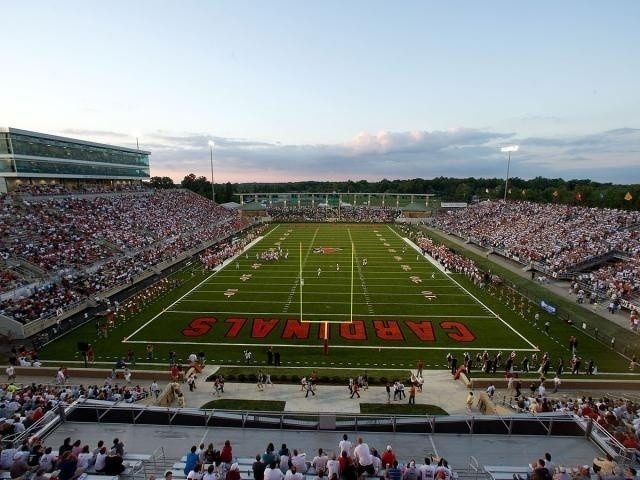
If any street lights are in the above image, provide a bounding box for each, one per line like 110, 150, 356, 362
500, 145, 519, 203
206, 139, 216, 202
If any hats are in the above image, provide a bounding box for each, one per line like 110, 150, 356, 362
386, 445, 393, 452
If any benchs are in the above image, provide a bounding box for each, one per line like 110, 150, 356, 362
155, 455, 329, 480
361, 462, 454, 480
484, 464, 601, 480
2, 449, 165, 480
